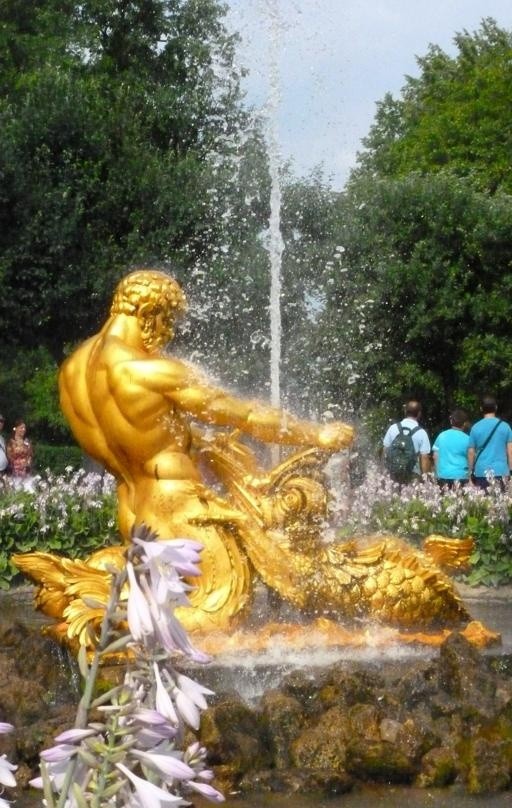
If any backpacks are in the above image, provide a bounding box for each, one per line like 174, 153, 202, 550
385, 422, 423, 483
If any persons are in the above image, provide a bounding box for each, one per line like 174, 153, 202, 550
17, 265, 352, 639
467, 394, 512, 493
0, 414, 10, 474
380, 398, 430, 486
430, 410, 472, 490
7, 420, 36, 476
